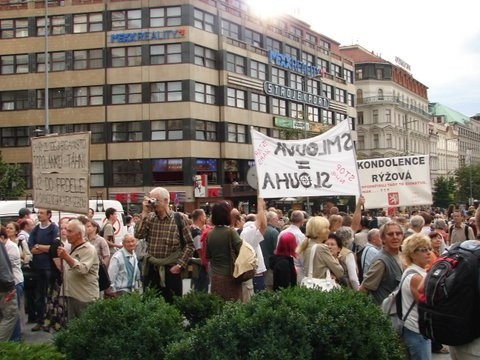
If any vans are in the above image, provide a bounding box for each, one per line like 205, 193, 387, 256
0, 198, 127, 291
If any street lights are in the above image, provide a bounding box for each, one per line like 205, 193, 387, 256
405, 114, 418, 155
302, 73, 321, 216
445, 137, 458, 190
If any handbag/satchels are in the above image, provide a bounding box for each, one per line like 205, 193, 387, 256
180, 257, 199, 279
300, 243, 336, 293
98, 264, 110, 291
229, 229, 254, 282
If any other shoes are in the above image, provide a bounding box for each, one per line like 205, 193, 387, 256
28, 314, 38, 323
31, 325, 41, 331
438, 347, 449, 354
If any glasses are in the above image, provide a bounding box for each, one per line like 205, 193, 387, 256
385, 231, 403, 236
416, 248, 432, 252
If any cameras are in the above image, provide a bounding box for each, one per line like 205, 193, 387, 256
147, 198, 156, 205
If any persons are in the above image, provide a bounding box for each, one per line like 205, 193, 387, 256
230, 198, 310, 291
205, 200, 258, 302
105, 234, 142, 298
301, 193, 406, 305
403, 202, 480, 272
134, 187, 196, 304
381, 233, 432, 360
99, 207, 123, 257
418, 203, 480, 360
88, 208, 94, 218
0, 207, 61, 345
57, 215, 110, 320
121, 212, 146, 236
181, 209, 215, 293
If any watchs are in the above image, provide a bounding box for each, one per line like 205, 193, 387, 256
35, 244, 39, 249
177, 264, 182, 268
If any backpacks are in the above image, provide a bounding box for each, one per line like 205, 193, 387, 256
382, 270, 422, 336
424, 240, 480, 346
